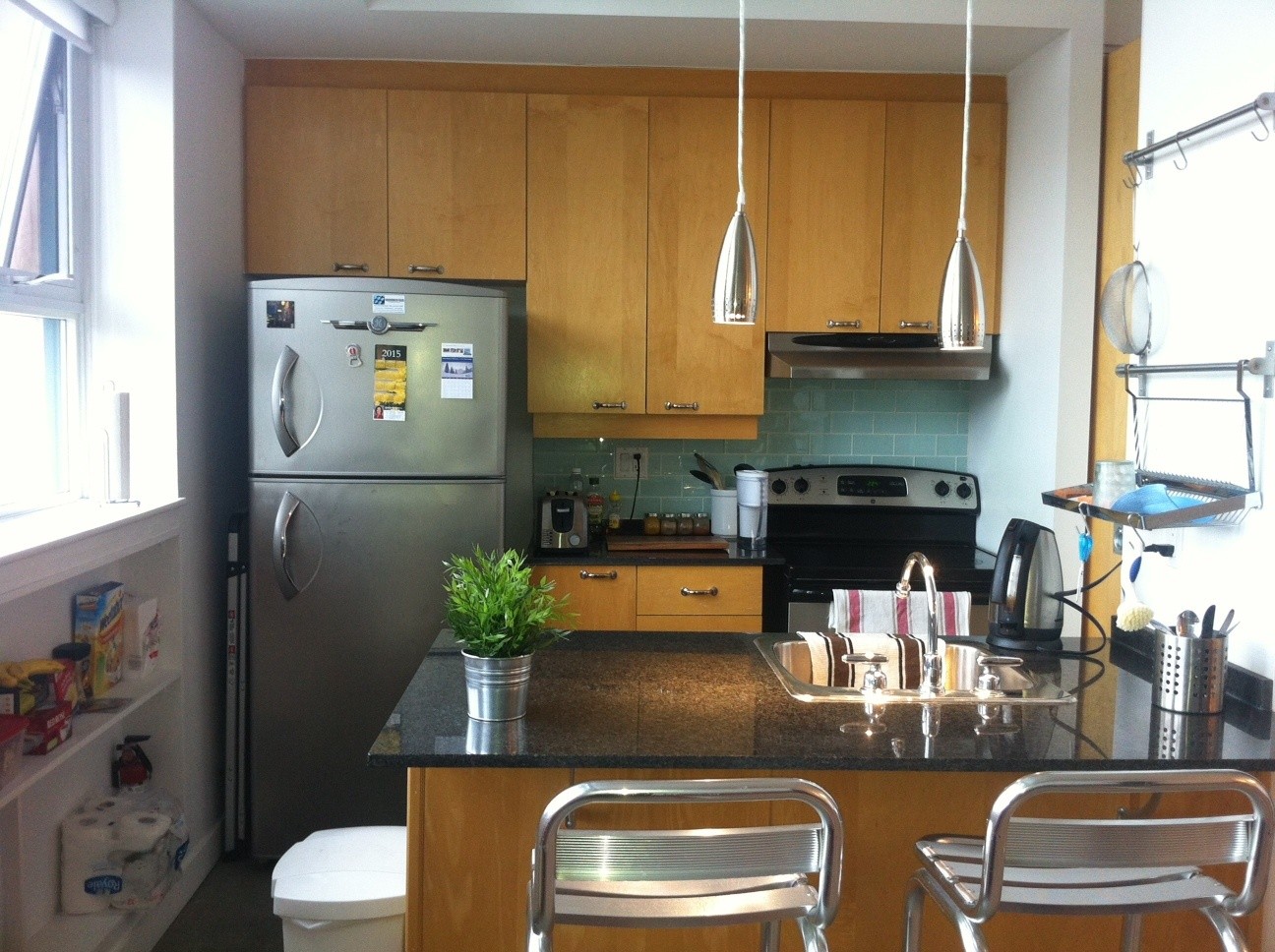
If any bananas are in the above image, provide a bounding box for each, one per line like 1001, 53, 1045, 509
0, 660, 66, 690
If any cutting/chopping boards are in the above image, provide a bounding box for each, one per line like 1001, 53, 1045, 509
607, 536, 729, 551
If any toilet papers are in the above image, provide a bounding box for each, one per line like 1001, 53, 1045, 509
58, 794, 171, 915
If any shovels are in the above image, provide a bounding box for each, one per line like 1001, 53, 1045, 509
695, 453, 723, 490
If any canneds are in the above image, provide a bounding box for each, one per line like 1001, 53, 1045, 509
645, 512, 709, 536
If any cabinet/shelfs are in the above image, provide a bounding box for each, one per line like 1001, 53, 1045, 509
0, 668, 183, 952
635, 566, 764, 633
764, 71, 1006, 334
243, 58, 527, 284
525, 65, 769, 440
521, 565, 636, 632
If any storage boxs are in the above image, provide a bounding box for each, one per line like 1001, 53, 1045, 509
123, 592, 160, 682
24, 700, 73, 756
0, 714, 30, 789
75, 581, 124, 699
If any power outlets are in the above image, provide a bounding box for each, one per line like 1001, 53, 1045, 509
1164, 527, 1183, 562
613, 447, 648, 479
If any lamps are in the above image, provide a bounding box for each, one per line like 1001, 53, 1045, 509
937, 1, 985, 351
711, 0, 759, 325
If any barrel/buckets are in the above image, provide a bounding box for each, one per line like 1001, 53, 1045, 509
460, 648, 535, 721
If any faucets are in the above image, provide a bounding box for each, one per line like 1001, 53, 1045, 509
896, 552, 945, 698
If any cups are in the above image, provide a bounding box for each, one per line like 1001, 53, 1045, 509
65, 715, 73, 728
710, 487, 737, 538
1091, 461, 1136, 509
59, 724, 70, 742
11, 739, 31, 754
25, 732, 45, 748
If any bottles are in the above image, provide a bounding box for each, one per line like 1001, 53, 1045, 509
660, 513, 676, 535
606, 489, 622, 536
643, 513, 660, 535
569, 468, 583, 495
52, 643, 95, 712
676, 513, 694, 535
586, 478, 604, 536
693, 513, 710, 535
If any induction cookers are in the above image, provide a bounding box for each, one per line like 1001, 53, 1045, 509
763, 463, 998, 581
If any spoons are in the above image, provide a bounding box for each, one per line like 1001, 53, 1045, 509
1149, 610, 1200, 638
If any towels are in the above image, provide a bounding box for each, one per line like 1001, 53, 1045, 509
828, 588, 973, 636
796, 631, 946, 688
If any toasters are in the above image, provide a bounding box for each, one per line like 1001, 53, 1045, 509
539, 490, 589, 555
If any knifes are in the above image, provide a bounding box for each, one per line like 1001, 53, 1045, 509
1202, 605, 1234, 638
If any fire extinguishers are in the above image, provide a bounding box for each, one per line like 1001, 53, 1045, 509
111, 733, 154, 791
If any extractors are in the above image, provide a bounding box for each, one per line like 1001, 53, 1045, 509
766, 332, 992, 381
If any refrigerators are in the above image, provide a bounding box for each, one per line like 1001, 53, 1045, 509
248, 277, 506, 868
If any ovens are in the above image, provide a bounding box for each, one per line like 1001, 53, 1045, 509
762, 582, 990, 637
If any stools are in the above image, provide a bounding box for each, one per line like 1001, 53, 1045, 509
902, 767, 1274, 952
526, 778, 845, 952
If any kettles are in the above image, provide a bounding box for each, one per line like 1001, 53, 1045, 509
986, 518, 1064, 651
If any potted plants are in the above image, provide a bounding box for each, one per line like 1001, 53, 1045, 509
440, 541, 581, 721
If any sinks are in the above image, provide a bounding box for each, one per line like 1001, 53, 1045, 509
753, 633, 1078, 704
